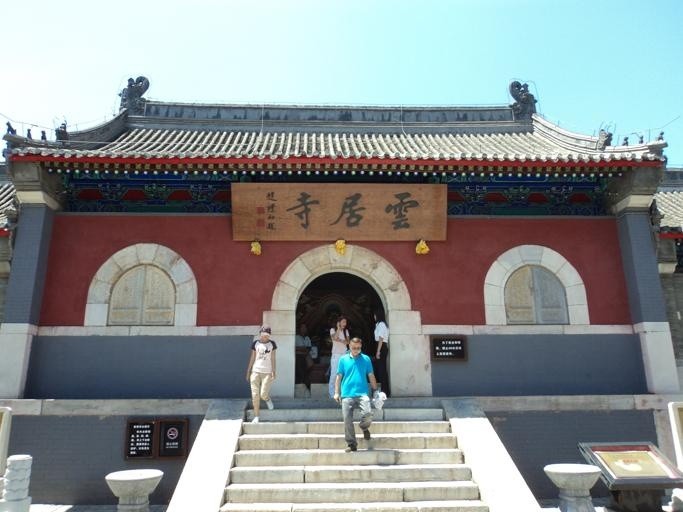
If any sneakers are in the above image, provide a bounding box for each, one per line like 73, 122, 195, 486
363, 430, 370, 440
266, 399, 273, 409
345, 443, 357, 451
251, 416, 259, 423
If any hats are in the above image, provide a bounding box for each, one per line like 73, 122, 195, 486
260, 326, 270, 336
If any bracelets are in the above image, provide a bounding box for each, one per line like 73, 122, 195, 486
374, 389, 378, 391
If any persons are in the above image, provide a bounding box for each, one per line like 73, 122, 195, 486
328, 315, 350, 400
373, 308, 389, 397
246, 327, 277, 423
334, 337, 379, 453
296, 323, 312, 390
